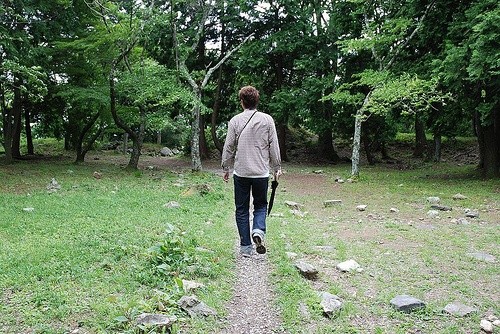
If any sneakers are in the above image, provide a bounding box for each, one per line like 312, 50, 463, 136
252, 232, 267, 254
240, 244, 252, 258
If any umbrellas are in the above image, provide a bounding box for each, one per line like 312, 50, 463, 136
268, 170, 279, 216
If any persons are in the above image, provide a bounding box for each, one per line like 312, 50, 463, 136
221, 85, 281, 256
